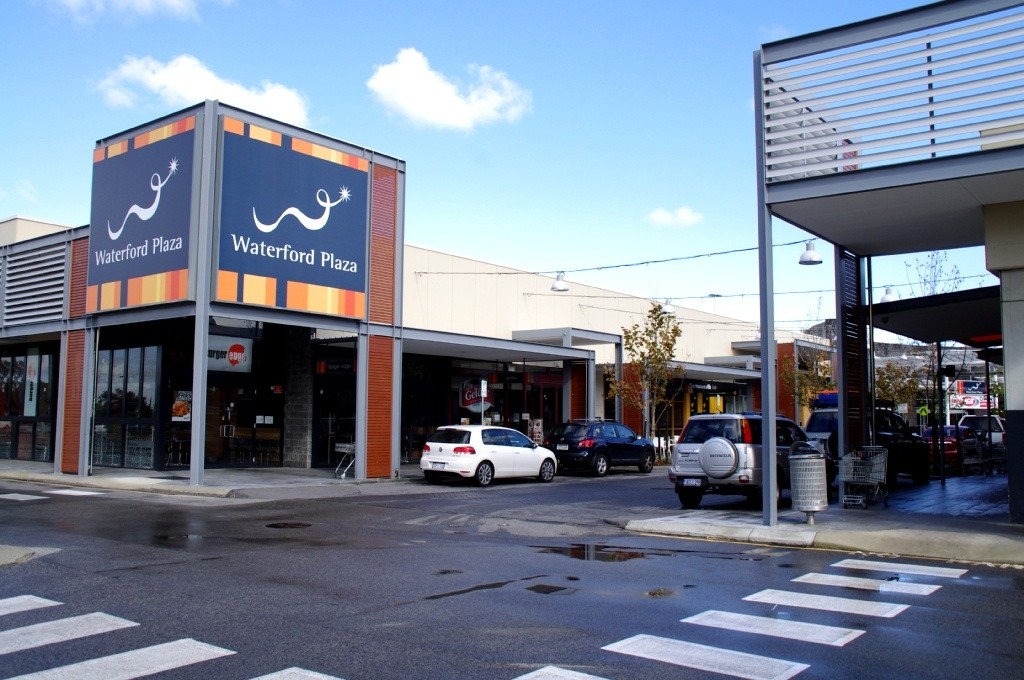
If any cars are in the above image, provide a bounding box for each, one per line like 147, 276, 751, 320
419, 424, 557, 486
922, 425, 986, 465
958, 414, 1005, 452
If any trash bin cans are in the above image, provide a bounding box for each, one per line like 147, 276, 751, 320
789, 440, 828, 512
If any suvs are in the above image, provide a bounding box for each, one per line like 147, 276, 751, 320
802, 396, 931, 487
669, 411, 814, 506
542, 418, 657, 478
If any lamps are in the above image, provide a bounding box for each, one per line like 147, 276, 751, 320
899, 352, 908, 359
755, 331, 762, 340
660, 299, 675, 314
798, 240, 822, 265
881, 287, 899, 302
551, 273, 569, 291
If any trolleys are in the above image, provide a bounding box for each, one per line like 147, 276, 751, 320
333, 444, 355, 479
